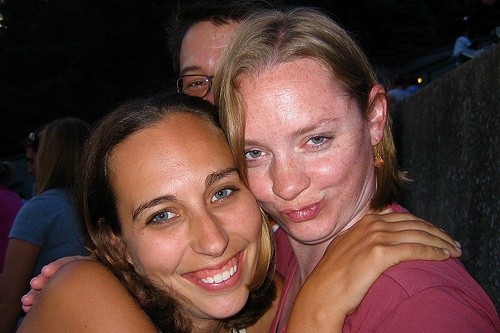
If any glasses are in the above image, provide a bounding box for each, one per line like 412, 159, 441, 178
177, 74, 214, 98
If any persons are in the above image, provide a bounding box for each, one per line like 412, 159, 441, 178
20, 6, 500, 333
174, 5, 253, 107
0, 118, 98, 333
17, 91, 464, 333
